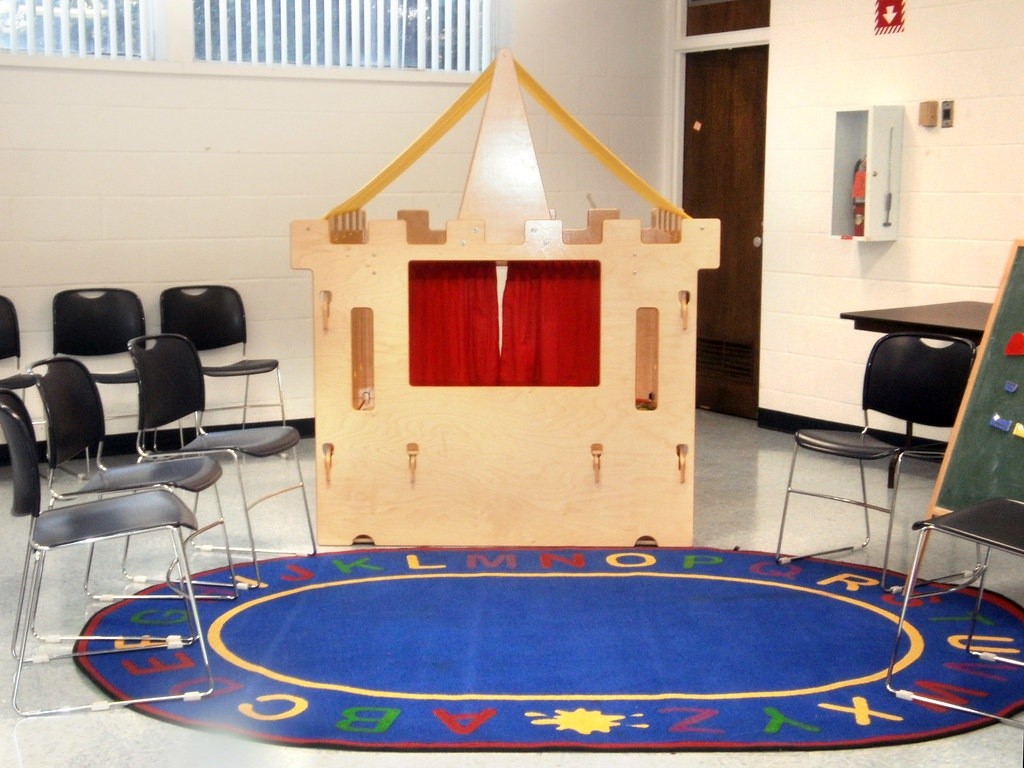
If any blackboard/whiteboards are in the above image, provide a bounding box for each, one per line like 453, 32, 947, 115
929, 236, 1024, 518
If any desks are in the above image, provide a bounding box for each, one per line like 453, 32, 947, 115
839, 300, 993, 508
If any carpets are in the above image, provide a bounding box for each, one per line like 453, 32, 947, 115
72, 546, 1024, 755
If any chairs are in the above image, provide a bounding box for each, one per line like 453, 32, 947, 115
884, 496, 1024, 729
159, 284, 296, 462
52, 288, 157, 482
774, 330, 983, 593
0, 389, 213, 718
0, 296, 57, 485
24, 357, 238, 642
120, 333, 317, 592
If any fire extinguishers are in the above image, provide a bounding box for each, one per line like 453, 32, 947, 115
853, 205, 865, 236
